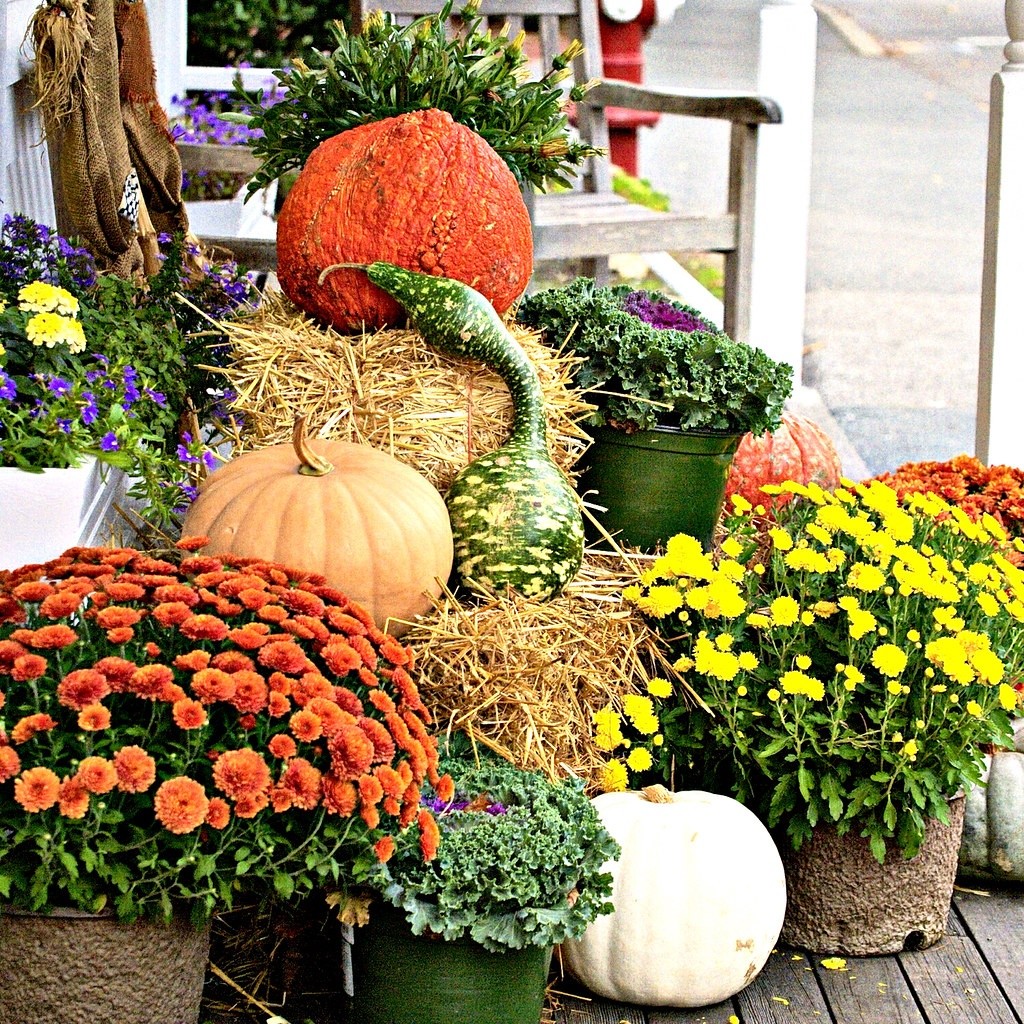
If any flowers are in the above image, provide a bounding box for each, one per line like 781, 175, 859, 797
0, 0, 1024, 932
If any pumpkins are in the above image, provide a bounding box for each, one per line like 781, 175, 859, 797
952, 716, 1024, 881
278, 106, 535, 335
552, 783, 786, 1007
717, 411, 841, 523
178, 410, 452, 636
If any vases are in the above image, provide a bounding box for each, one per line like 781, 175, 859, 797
774, 775, 967, 959
0, 894, 213, 1024
341, 878, 553, 1024
577, 411, 747, 552
177, 140, 268, 175
0, 437, 149, 586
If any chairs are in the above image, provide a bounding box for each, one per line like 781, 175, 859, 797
356, 0, 786, 348
